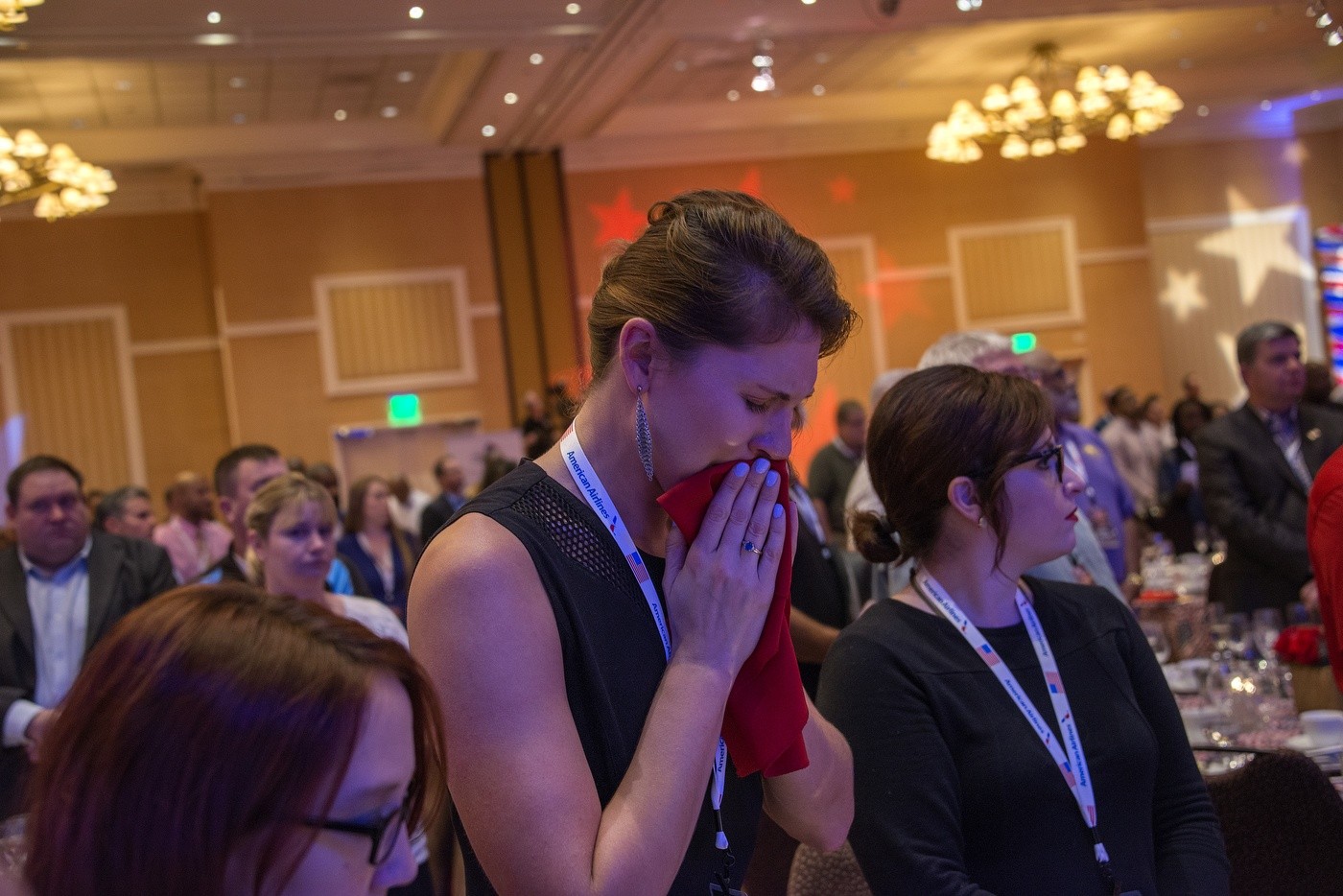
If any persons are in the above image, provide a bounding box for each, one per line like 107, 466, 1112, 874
13, 579, 432, 894
847, 328, 1136, 620
806, 397, 870, 539
0, 372, 585, 758
1016, 316, 1341, 613
398, 183, 867, 895
812, 363, 1222, 896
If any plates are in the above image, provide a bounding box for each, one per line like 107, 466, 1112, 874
1288, 735, 1342, 756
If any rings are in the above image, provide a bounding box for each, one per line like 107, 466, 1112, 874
741, 540, 762, 556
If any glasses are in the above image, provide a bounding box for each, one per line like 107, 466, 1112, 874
254, 780, 421, 866
930, 444, 1065, 512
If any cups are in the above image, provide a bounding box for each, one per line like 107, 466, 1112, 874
1136, 543, 1302, 777
1301, 708, 1341, 747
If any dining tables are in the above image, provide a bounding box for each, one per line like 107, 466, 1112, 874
1139, 643, 1343, 796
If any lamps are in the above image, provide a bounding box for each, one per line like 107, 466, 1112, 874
0, 125, 122, 225
920, 45, 1185, 167
